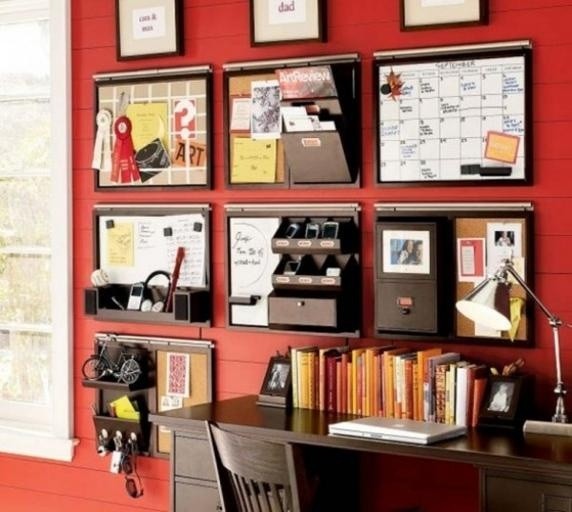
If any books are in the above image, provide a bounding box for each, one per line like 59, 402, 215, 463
291, 345, 492, 430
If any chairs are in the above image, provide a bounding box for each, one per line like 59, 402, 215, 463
204, 417, 305, 512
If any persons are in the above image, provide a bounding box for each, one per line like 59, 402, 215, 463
271, 363, 283, 391
397, 241, 414, 264
487, 383, 508, 412
497, 231, 510, 246
408, 241, 422, 264
253, 88, 276, 118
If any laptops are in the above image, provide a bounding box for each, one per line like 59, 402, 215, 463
328, 416, 466, 446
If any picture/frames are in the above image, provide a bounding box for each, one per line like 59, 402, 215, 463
255, 355, 291, 397
479, 370, 520, 420
246, 0, 330, 46
369, 198, 539, 348
150, 342, 213, 461
371, 38, 536, 188
398, 0, 490, 33
220, 200, 367, 341
90, 200, 215, 330
220, 51, 366, 192
88, 62, 218, 194
112, 0, 185, 60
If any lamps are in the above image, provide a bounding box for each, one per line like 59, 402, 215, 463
451, 256, 572, 437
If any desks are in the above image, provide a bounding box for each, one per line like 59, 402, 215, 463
148, 396, 572, 512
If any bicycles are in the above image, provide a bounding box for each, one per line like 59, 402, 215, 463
81, 335, 142, 388
